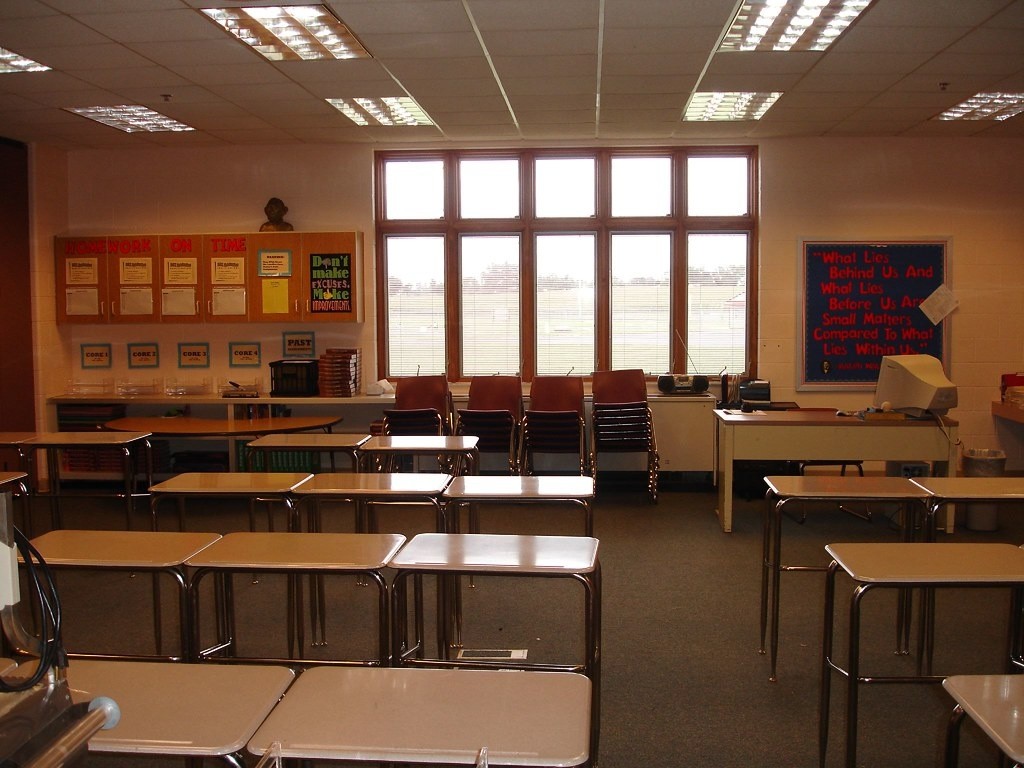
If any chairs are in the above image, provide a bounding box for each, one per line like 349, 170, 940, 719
775, 408, 871, 524
370, 368, 659, 504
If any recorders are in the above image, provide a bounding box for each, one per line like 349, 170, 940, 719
657, 329, 710, 394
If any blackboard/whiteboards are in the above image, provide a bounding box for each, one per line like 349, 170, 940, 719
798, 238, 951, 388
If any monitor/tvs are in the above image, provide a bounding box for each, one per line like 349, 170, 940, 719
873, 354, 958, 419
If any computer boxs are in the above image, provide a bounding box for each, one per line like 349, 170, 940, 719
882, 460, 931, 527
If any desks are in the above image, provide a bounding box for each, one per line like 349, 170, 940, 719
759, 476, 1024, 768
713, 408, 959, 534
0, 431, 602, 768
52, 393, 718, 495
106, 416, 342, 508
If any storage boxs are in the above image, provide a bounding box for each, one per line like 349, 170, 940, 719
63, 376, 264, 395
269, 348, 362, 398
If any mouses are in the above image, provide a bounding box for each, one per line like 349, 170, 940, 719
836, 411, 852, 416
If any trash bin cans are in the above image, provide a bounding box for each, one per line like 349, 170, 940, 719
961, 447, 1008, 532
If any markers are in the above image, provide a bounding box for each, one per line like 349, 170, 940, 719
229, 381, 246, 391
